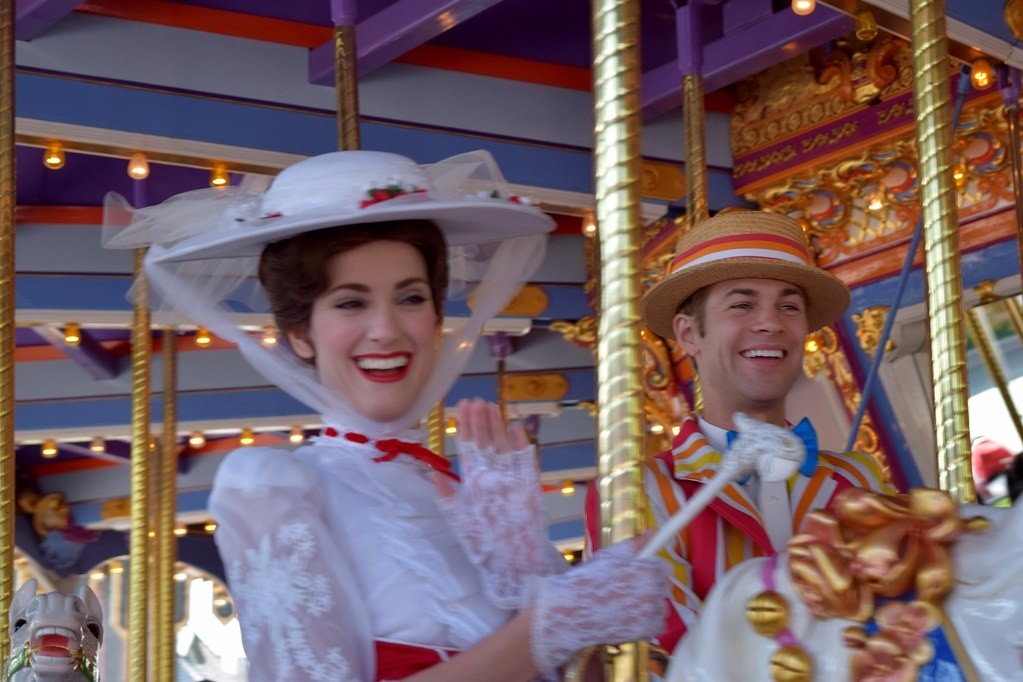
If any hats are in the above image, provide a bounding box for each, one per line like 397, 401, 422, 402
149, 150, 556, 267
641, 212, 851, 343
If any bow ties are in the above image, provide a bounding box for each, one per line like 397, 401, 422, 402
725, 417, 818, 484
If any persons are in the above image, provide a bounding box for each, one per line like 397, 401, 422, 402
207, 151, 674, 682
584, 210, 982, 682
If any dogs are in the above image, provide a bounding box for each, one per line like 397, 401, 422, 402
6, 579, 105, 681
